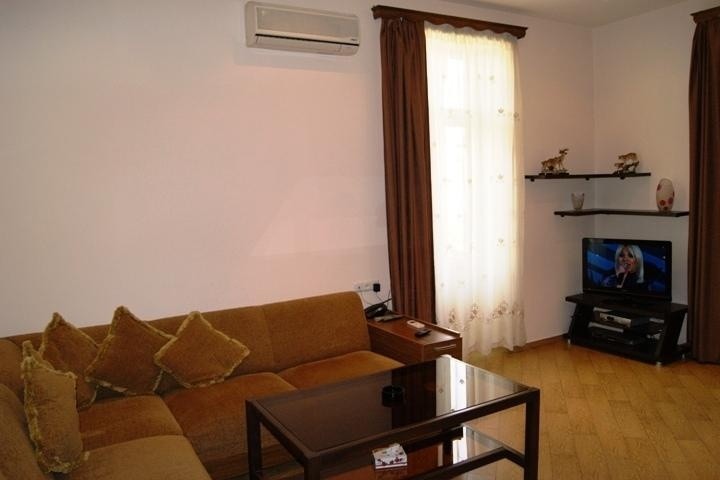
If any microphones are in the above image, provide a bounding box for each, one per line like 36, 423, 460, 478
617, 264, 628, 288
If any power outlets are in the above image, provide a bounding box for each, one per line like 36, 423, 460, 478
354, 280, 379, 292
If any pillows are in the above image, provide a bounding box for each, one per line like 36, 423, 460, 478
20, 306, 250, 472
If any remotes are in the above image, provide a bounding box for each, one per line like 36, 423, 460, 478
415, 329, 431, 337
408, 320, 425, 328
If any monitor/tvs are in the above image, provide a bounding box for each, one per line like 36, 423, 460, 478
582, 237, 672, 304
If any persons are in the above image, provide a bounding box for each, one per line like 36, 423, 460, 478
600, 243, 656, 291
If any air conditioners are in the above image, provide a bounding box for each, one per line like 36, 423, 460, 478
246, 0, 360, 57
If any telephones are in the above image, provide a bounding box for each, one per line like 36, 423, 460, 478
364, 297, 393, 319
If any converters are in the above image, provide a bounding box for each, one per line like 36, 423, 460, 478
373, 283, 380, 292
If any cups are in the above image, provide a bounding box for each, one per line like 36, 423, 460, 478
571, 192, 585, 209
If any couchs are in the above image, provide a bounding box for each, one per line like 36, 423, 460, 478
0, 290, 403, 480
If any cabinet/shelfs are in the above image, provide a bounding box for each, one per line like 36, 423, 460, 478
367, 312, 462, 367
563, 297, 688, 365
525, 172, 690, 217
